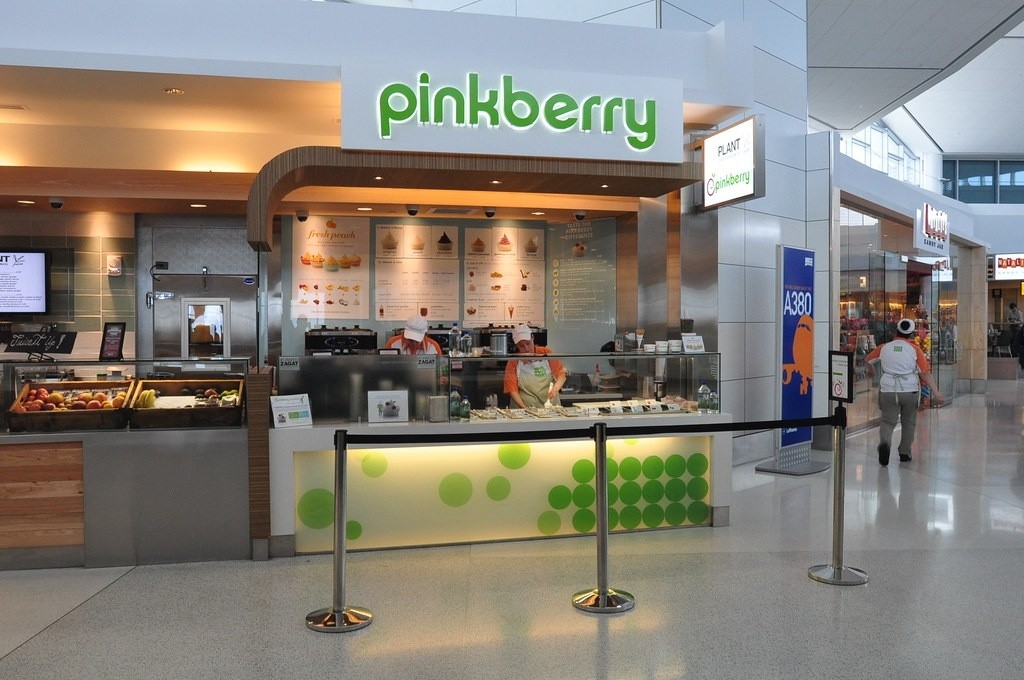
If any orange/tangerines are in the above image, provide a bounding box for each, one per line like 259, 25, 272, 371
102, 391, 126, 408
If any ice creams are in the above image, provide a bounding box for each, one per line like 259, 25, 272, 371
508, 304, 514, 320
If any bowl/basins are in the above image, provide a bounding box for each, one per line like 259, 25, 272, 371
643, 344, 656, 355
668, 339, 682, 353
655, 341, 668, 354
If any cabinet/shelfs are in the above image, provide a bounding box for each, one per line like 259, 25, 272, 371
841, 329, 872, 394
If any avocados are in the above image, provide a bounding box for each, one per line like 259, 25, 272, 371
154, 387, 204, 408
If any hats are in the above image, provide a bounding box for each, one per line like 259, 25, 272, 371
512, 325, 532, 343
404, 315, 428, 343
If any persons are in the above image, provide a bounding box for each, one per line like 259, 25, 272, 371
1007, 303, 1024, 357
503, 325, 567, 409
385, 314, 450, 389
864, 319, 944, 466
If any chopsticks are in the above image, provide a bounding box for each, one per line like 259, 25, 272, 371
681, 319, 694, 333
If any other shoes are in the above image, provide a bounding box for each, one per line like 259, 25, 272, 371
898, 454, 912, 462
879, 442, 890, 465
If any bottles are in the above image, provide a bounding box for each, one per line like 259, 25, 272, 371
451, 387, 462, 422
459, 395, 470, 421
697, 382, 711, 413
460, 330, 472, 357
449, 323, 461, 356
708, 389, 718, 414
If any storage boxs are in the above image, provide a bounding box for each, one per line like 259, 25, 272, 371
7, 380, 135, 431
127, 380, 244, 431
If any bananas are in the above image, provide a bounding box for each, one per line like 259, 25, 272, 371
138, 388, 155, 408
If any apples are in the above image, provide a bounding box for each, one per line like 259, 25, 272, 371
22, 388, 105, 411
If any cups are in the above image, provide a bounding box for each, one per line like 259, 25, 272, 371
654, 381, 664, 399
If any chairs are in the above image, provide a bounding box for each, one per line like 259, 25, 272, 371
992, 330, 1012, 357
